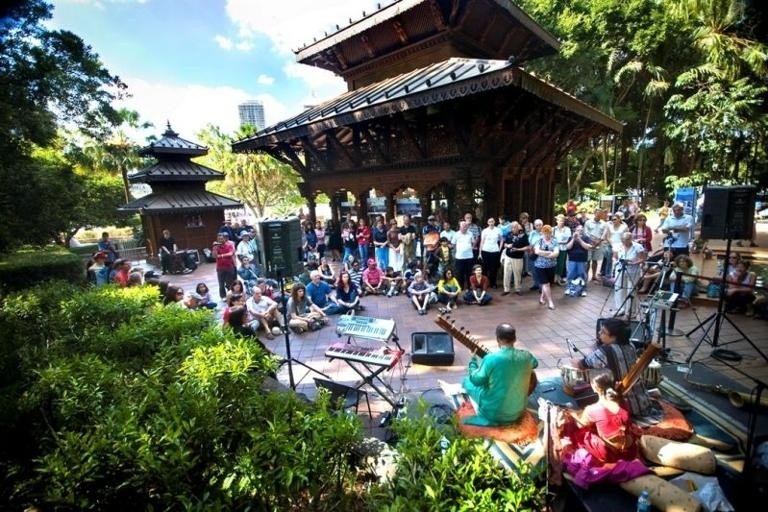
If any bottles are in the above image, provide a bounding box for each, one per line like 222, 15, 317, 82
728, 265, 738, 280
636, 491, 652, 511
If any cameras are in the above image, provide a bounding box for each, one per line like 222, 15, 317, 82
618, 259, 625, 263
215, 243, 223, 246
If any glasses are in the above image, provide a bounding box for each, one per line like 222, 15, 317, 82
175, 291, 184, 297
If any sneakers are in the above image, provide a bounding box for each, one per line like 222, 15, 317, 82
678, 301, 691, 309
498, 273, 602, 297
222, 289, 429, 340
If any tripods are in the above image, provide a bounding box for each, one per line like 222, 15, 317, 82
599, 264, 643, 319
631, 262, 712, 358
686, 240, 768, 363
272, 278, 331, 391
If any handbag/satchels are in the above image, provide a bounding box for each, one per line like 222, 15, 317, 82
527, 244, 539, 262
706, 280, 729, 298
500, 249, 509, 265
569, 277, 586, 298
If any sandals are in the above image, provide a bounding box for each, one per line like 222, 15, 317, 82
438, 306, 448, 315
538, 296, 556, 311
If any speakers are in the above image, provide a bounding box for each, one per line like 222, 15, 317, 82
596, 318, 653, 348
700, 184, 756, 240
258, 216, 305, 279
409, 332, 454, 364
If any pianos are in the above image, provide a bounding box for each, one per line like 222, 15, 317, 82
322, 313, 406, 368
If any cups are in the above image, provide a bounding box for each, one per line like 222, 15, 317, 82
715, 259, 722, 269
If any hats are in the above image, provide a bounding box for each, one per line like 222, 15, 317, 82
240, 230, 250, 238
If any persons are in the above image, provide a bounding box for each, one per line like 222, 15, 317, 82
229, 305, 277, 381
92, 229, 218, 309
569, 315, 649, 413
468, 322, 538, 422
639, 200, 699, 308
212, 200, 652, 332
712, 249, 756, 313
566, 367, 636, 463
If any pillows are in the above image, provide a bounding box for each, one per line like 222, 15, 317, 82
621, 397, 692, 441
641, 435, 716, 475
648, 464, 684, 476
456, 402, 536, 445
685, 433, 732, 450
712, 451, 745, 474
621, 470, 702, 511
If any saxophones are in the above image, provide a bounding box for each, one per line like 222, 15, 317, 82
684, 359, 768, 408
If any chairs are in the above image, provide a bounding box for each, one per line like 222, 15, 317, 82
314, 377, 373, 420
